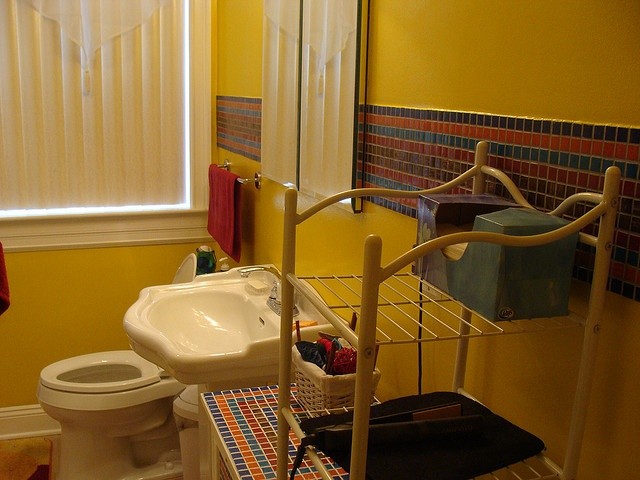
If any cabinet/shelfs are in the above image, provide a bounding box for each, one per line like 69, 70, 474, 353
277, 140, 621, 478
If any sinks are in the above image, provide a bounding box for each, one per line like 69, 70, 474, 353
139, 280, 272, 366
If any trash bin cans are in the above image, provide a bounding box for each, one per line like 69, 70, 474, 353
173, 383, 199, 480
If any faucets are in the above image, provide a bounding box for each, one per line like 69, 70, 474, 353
238, 266, 300, 319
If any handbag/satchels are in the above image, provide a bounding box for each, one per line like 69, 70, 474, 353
297, 391, 546, 480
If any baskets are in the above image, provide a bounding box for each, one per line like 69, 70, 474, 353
292, 337, 381, 419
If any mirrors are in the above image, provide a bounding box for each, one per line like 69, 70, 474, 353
0, 1, 211, 255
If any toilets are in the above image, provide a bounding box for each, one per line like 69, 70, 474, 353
37, 253, 198, 480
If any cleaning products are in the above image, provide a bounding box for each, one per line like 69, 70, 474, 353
219, 256, 230, 270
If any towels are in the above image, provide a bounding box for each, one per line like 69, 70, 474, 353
0, 242, 10, 314
206, 163, 242, 263
